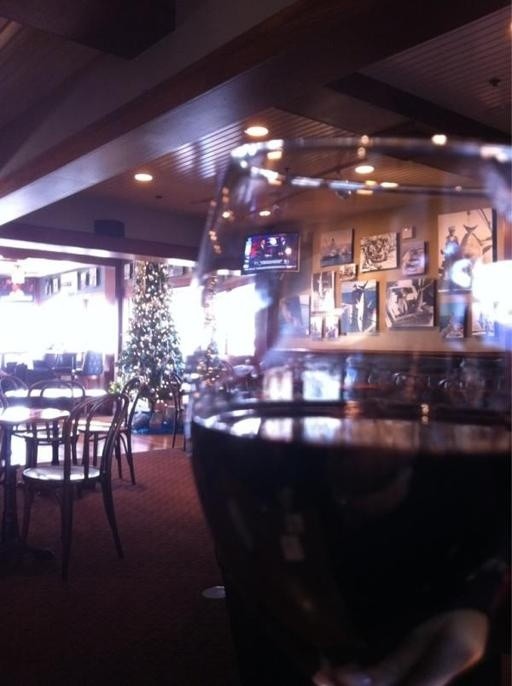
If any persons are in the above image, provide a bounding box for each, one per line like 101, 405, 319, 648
446, 226, 459, 255
249, 236, 288, 259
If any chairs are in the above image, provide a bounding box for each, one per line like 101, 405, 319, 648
163, 369, 188, 452
0, 351, 142, 582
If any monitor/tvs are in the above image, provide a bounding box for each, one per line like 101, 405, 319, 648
241, 231, 300, 274
